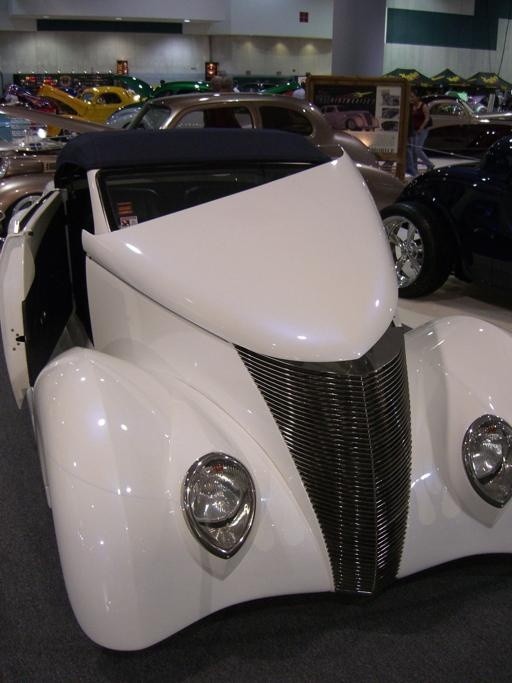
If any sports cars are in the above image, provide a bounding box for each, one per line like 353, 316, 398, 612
0, 120, 512, 652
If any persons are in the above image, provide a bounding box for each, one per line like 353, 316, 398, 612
202, 75, 241, 128
58, 77, 77, 96
409, 88, 435, 172
494, 90, 512, 112
382, 100, 418, 177
292, 81, 306, 100
305, 72, 311, 78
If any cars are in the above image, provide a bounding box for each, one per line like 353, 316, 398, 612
380, 92, 511, 309
